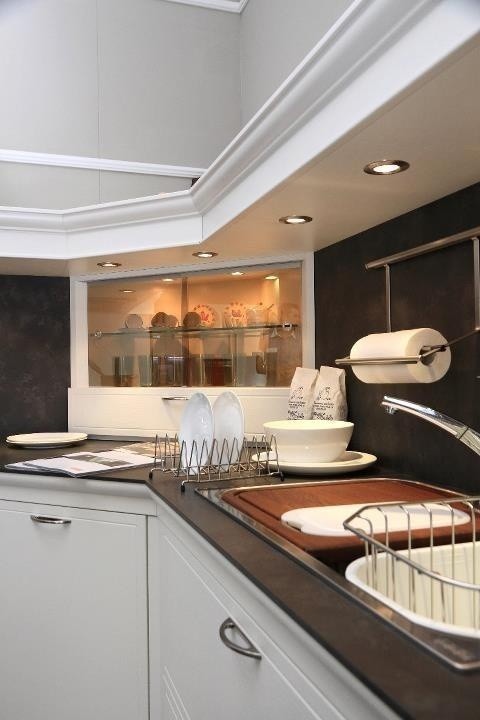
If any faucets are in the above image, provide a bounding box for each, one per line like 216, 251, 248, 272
380, 394, 480, 455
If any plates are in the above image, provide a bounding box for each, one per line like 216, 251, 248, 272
250, 446, 379, 478
5, 432, 89, 450
177, 392, 217, 475
212, 389, 245, 474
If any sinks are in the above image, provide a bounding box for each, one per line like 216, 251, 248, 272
307, 532, 480, 673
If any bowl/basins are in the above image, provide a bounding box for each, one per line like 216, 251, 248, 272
263, 418, 355, 464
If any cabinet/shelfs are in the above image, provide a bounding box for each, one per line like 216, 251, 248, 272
0, 472, 148, 720
149, 484, 407, 720
67, 252, 316, 448
